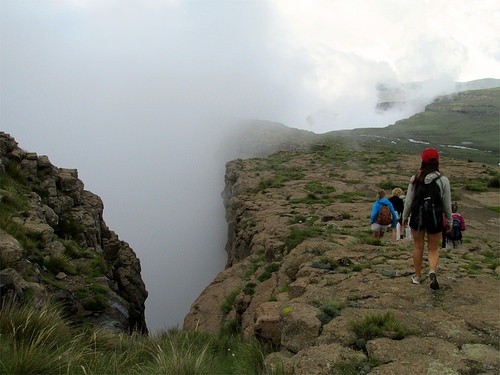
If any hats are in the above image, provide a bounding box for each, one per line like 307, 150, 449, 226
420, 147, 439, 164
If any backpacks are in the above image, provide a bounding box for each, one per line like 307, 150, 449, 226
377, 204, 393, 225
446, 218, 462, 246
408, 173, 444, 230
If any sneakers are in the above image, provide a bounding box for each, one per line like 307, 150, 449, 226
429, 271, 439, 290
411, 274, 421, 285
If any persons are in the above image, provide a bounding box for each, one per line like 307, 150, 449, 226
370, 188, 405, 240
447, 202, 466, 248
402, 148, 452, 289
